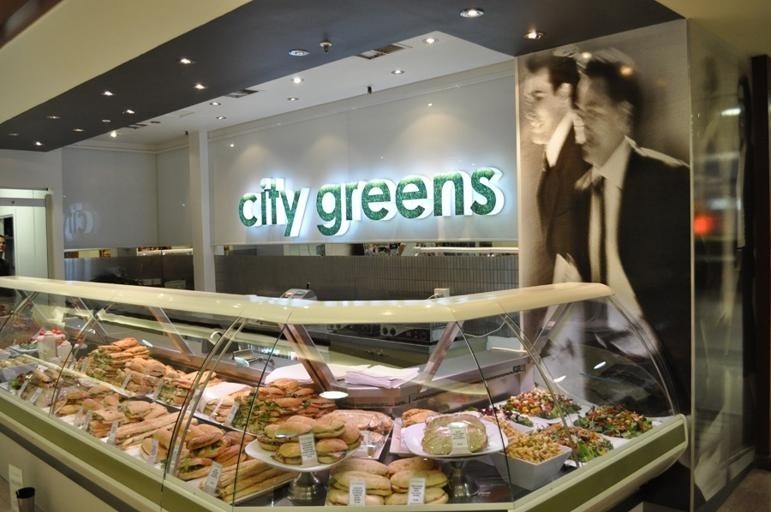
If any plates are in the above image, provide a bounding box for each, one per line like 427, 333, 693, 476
245, 427, 365, 470
480, 391, 666, 467
404, 416, 510, 457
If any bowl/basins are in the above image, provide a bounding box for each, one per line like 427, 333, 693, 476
493, 442, 574, 491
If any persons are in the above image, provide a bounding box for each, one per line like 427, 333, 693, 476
563, 49, 692, 420
694, 53, 725, 291
0, 233, 12, 299
524, 51, 588, 397
731, 70, 767, 452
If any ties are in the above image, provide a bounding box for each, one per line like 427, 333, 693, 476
592, 176, 605, 285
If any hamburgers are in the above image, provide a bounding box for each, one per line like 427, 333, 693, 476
0, 338, 363, 480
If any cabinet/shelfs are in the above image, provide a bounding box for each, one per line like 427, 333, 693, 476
0, 274, 691, 512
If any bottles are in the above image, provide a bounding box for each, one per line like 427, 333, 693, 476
38, 327, 66, 362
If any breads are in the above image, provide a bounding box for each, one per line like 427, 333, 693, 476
402, 408, 440, 426
327, 457, 450, 506
200, 458, 297, 501
423, 414, 487, 454
335, 408, 393, 454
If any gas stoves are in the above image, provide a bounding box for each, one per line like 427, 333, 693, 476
331, 322, 468, 348
